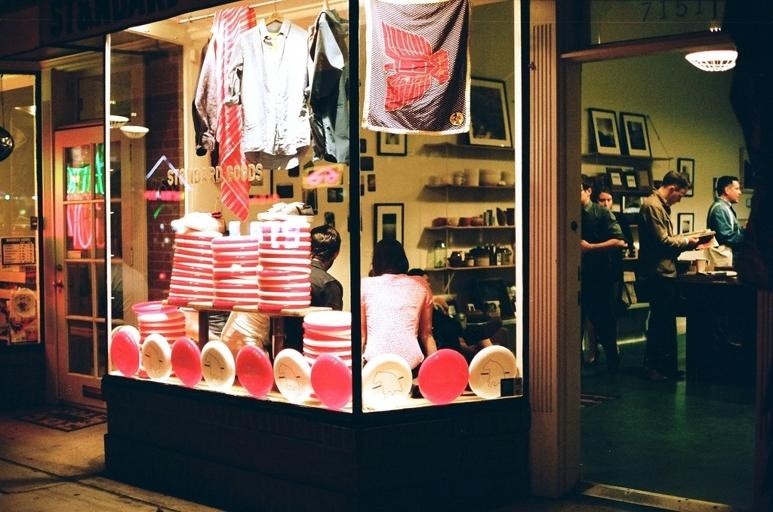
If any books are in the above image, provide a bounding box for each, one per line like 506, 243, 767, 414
677, 228, 720, 250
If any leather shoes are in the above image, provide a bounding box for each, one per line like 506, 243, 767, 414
462, 316, 503, 346
664, 369, 685, 378
617, 344, 624, 362
585, 351, 600, 366
641, 367, 669, 382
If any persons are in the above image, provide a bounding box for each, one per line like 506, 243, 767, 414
208, 311, 229, 336
361, 239, 437, 379
579, 176, 628, 377
459, 311, 503, 354
407, 268, 464, 349
729, 0, 773, 287
585, 186, 633, 366
220, 313, 270, 376
282, 226, 343, 351
638, 171, 699, 382
706, 176, 745, 267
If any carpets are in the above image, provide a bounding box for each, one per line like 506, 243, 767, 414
15, 405, 108, 431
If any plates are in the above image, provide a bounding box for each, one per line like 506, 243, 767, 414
211, 236, 258, 306
417, 349, 469, 405
361, 353, 412, 413
235, 345, 274, 396
467, 345, 517, 400
199, 341, 235, 391
130, 300, 185, 349
166, 229, 221, 303
249, 220, 311, 313
110, 330, 140, 377
302, 309, 351, 372
171, 337, 202, 387
310, 354, 351, 409
141, 334, 172, 381
273, 349, 311, 404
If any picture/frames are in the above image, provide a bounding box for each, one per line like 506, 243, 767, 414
607, 168, 626, 188
249, 169, 272, 198
678, 213, 694, 235
375, 203, 403, 247
625, 173, 638, 188
588, 108, 622, 155
469, 77, 513, 147
677, 158, 695, 196
620, 112, 651, 157
622, 194, 640, 213
739, 146, 758, 194
377, 132, 407, 155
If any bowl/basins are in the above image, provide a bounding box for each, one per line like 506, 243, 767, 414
478, 168, 501, 186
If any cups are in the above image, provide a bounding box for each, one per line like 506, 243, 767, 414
429, 177, 442, 187
695, 259, 710, 273
442, 176, 454, 185
460, 218, 473, 227
454, 171, 467, 186
462, 166, 480, 188
434, 218, 448, 227
448, 217, 459, 226
473, 217, 485, 226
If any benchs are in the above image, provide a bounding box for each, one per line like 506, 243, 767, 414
163, 299, 331, 391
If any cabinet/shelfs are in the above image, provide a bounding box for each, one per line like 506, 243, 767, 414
104, 371, 526, 512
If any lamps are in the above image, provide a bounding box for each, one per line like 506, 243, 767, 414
683, 0, 739, 72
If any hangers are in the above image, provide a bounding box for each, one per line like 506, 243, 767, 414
238, 0, 309, 41
308, 0, 348, 66
